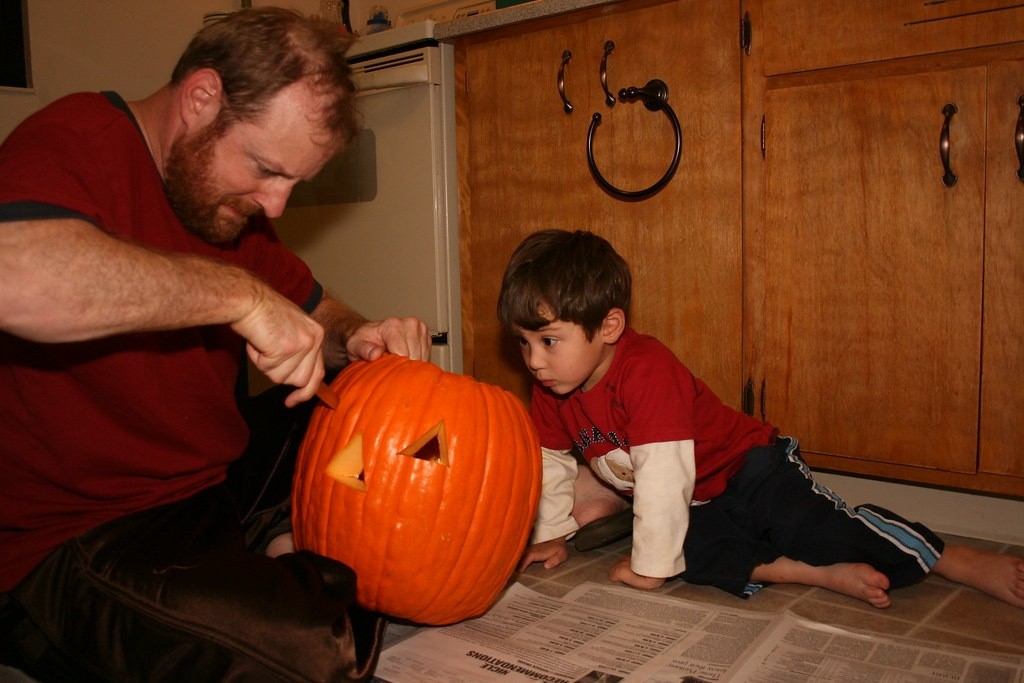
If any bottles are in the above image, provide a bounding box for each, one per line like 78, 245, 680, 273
365, 19, 390, 35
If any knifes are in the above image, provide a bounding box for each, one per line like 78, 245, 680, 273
315, 382, 341, 412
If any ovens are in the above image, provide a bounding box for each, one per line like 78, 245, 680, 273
247, 19, 465, 397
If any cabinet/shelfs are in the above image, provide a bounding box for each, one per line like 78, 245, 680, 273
435, 0, 1024, 548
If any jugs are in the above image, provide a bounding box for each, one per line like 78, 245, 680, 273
319, 0, 353, 34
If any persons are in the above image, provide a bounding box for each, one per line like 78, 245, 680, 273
0, 6, 431, 683
495, 228, 1024, 613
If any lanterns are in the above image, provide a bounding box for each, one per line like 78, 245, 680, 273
290, 350, 542, 626
